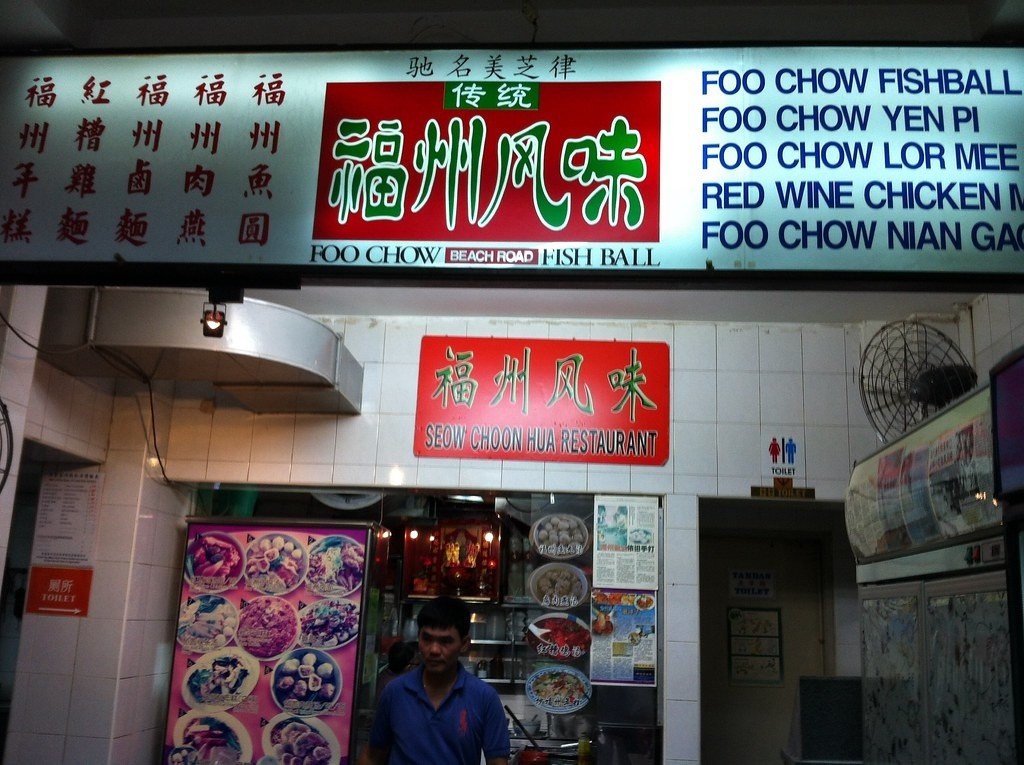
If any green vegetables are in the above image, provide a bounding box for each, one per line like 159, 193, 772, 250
175, 536, 344, 750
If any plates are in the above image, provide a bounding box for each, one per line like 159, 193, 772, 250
526, 665, 592, 714
634, 595, 655, 611
530, 563, 587, 610
169, 531, 364, 765
534, 514, 588, 558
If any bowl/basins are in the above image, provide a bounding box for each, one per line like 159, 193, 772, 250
527, 612, 591, 660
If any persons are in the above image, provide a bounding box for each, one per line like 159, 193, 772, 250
361, 595, 510, 765
377, 641, 414, 698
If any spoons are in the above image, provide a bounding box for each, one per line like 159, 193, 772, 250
528, 623, 552, 644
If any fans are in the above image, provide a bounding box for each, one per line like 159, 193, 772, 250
856, 321, 978, 445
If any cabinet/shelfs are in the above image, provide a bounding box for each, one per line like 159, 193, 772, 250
398, 513, 539, 690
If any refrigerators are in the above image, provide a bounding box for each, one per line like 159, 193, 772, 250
844, 382, 1017, 765
